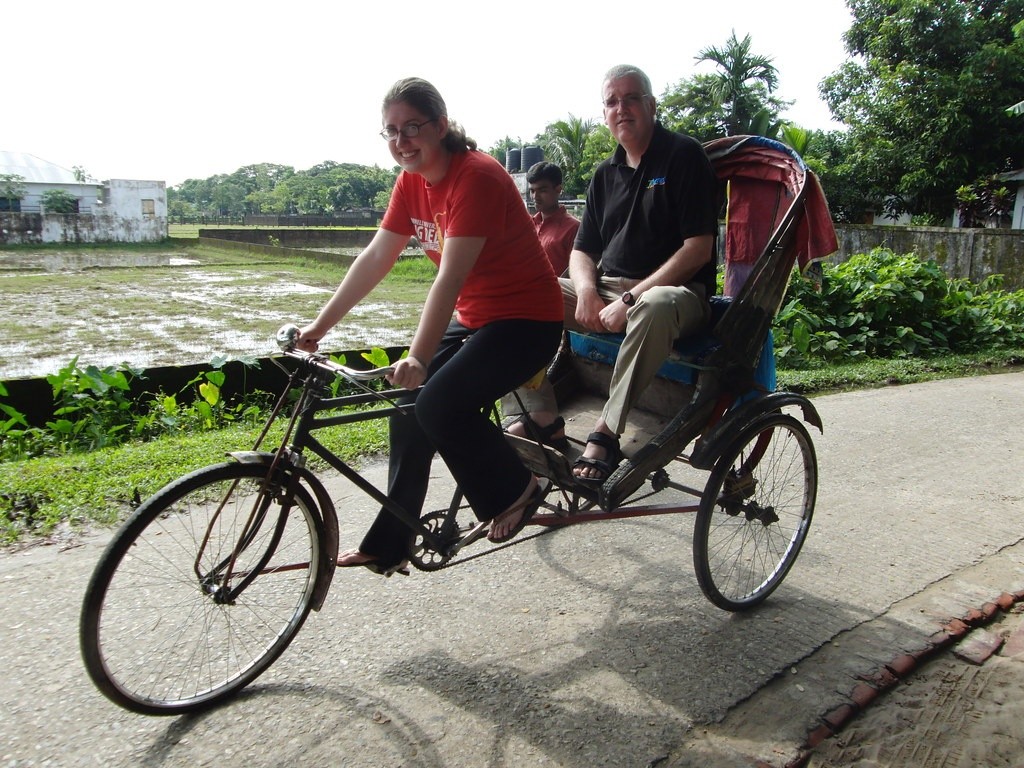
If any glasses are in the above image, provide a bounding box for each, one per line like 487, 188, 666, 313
380, 120, 434, 141
603, 92, 646, 107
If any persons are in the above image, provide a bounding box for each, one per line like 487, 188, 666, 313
290, 77, 549, 568
525, 162, 578, 277
501, 65, 719, 482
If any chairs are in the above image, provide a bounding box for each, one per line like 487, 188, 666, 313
564, 133, 809, 385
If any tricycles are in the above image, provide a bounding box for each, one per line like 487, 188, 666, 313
80, 136, 838, 712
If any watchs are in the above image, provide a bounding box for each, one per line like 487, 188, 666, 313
621, 290, 635, 307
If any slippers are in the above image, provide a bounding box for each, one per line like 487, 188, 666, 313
487, 478, 553, 543
334, 546, 386, 566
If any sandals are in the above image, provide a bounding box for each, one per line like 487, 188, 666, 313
500, 413, 567, 448
572, 424, 621, 482
